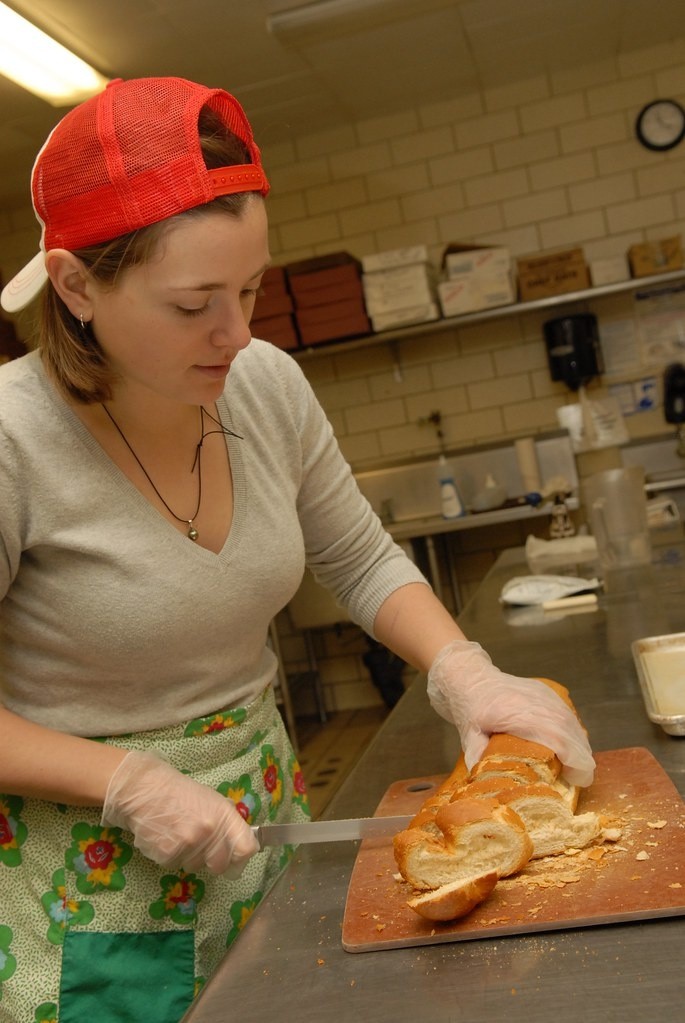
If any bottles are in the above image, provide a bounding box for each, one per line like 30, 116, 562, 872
437, 455, 465, 520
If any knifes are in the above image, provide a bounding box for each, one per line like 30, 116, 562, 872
249, 814, 418, 845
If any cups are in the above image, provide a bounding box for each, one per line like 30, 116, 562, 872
582, 465, 653, 573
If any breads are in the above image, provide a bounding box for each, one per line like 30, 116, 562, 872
391, 677, 621, 926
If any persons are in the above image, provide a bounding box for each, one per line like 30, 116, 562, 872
1, 77, 596, 1023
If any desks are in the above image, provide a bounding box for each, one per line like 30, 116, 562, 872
373, 491, 580, 614
177, 521, 685, 1023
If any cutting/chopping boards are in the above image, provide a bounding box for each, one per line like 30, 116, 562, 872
342, 746, 685, 953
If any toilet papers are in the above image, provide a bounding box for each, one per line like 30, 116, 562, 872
515, 438, 543, 491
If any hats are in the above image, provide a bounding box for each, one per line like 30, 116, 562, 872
1, 76, 270, 314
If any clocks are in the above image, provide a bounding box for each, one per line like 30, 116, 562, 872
636, 98, 685, 152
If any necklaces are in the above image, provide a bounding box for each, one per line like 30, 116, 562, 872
101, 403, 244, 540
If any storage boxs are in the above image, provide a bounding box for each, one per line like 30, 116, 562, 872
518, 248, 590, 305
254, 281, 286, 305
301, 315, 370, 345
627, 234, 685, 280
590, 255, 632, 289
363, 275, 436, 299
249, 313, 293, 338
371, 302, 441, 333
266, 329, 300, 350
293, 278, 361, 307
286, 250, 359, 292
255, 266, 282, 287
361, 263, 437, 285
296, 298, 363, 326
436, 271, 517, 317
438, 241, 512, 281
362, 242, 436, 272
365, 286, 438, 315
250, 295, 293, 324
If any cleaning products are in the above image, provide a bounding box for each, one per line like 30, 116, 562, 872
435, 453, 467, 520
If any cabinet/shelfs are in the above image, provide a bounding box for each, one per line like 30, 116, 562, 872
271, 604, 329, 755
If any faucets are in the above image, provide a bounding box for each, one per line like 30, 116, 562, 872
417, 417, 435, 430
